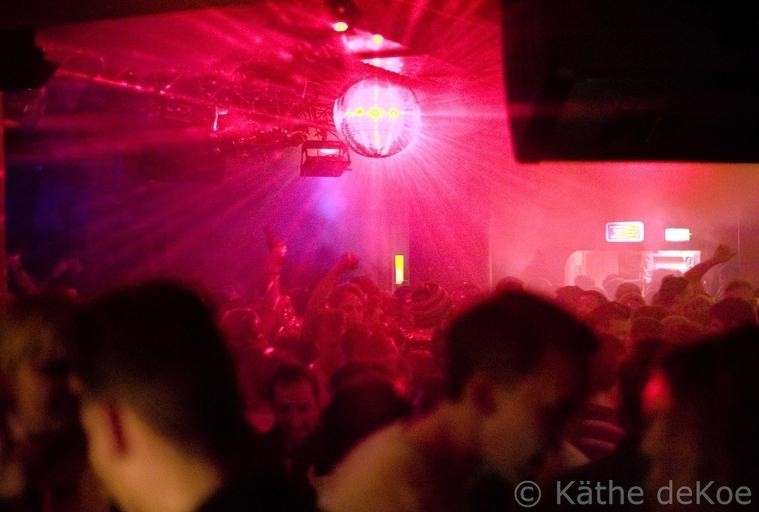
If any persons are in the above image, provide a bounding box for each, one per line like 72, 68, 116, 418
1, 233, 759, 510
